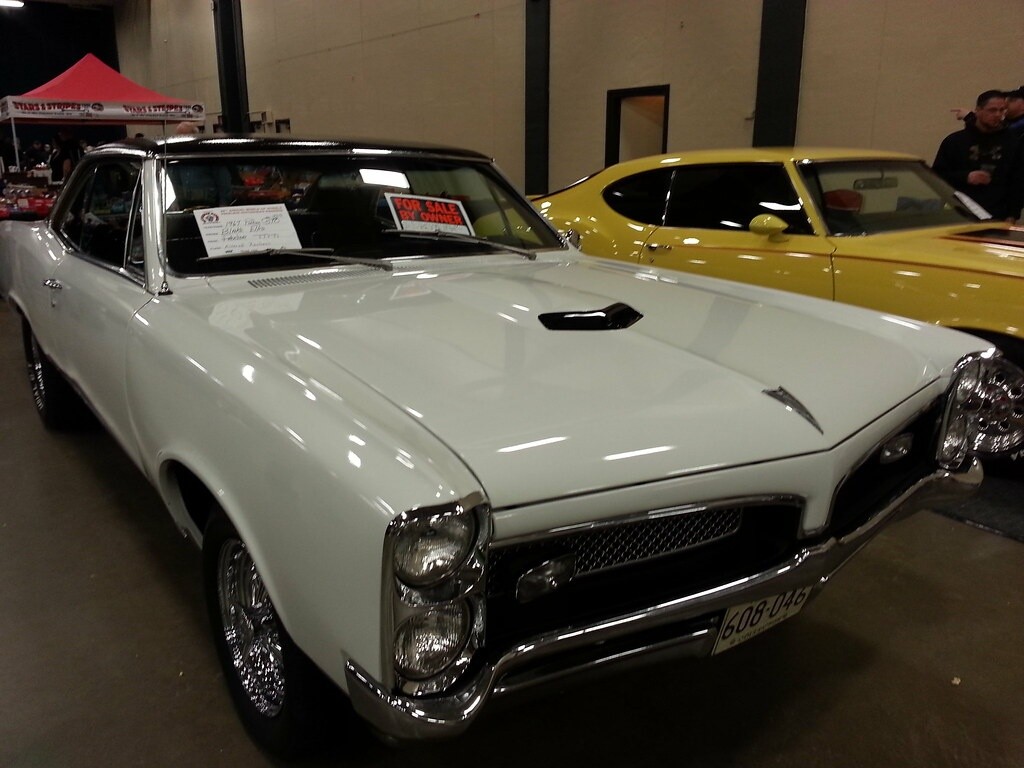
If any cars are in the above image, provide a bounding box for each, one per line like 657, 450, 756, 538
0, 134, 1024, 751
471, 144, 1024, 404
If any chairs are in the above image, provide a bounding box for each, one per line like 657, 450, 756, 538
824, 190, 863, 215
896, 196, 944, 211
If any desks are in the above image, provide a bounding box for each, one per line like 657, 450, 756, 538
0, 196, 55, 219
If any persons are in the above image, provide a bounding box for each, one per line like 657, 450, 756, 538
8, 131, 91, 186
176, 122, 200, 136
931, 85, 1024, 224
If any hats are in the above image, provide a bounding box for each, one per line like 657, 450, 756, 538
1004, 86, 1024, 100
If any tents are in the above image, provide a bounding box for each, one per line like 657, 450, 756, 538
0, 54, 205, 174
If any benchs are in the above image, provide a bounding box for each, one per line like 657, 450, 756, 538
90, 214, 200, 245
